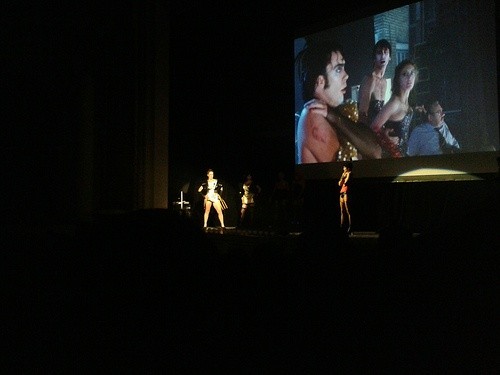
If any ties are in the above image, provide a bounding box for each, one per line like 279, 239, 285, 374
435, 128, 447, 150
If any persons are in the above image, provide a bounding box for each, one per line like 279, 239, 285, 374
197, 169, 227, 228
297, 34, 460, 163
238, 174, 261, 225
336, 162, 355, 232
